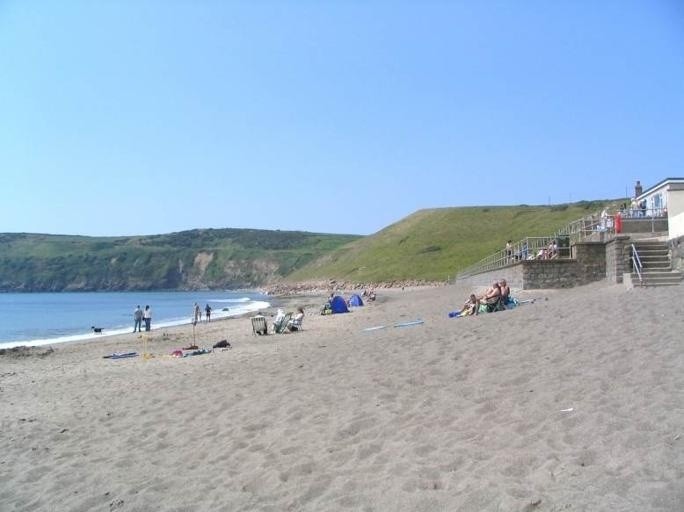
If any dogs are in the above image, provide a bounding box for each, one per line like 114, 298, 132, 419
91, 326, 105, 335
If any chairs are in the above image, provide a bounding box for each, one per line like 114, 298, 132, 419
251, 317, 268, 336
272, 313, 305, 335
480, 286, 509, 313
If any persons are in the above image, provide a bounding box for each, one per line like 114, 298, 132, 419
459, 279, 510, 316
506, 240, 557, 259
255, 312, 269, 336
193, 302, 202, 325
600, 206, 623, 234
133, 305, 151, 332
204, 303, 211, 323
287, 308, 304, 333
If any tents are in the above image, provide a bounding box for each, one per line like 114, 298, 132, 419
347, 294, 363, 306
328, 295, 349, 313
